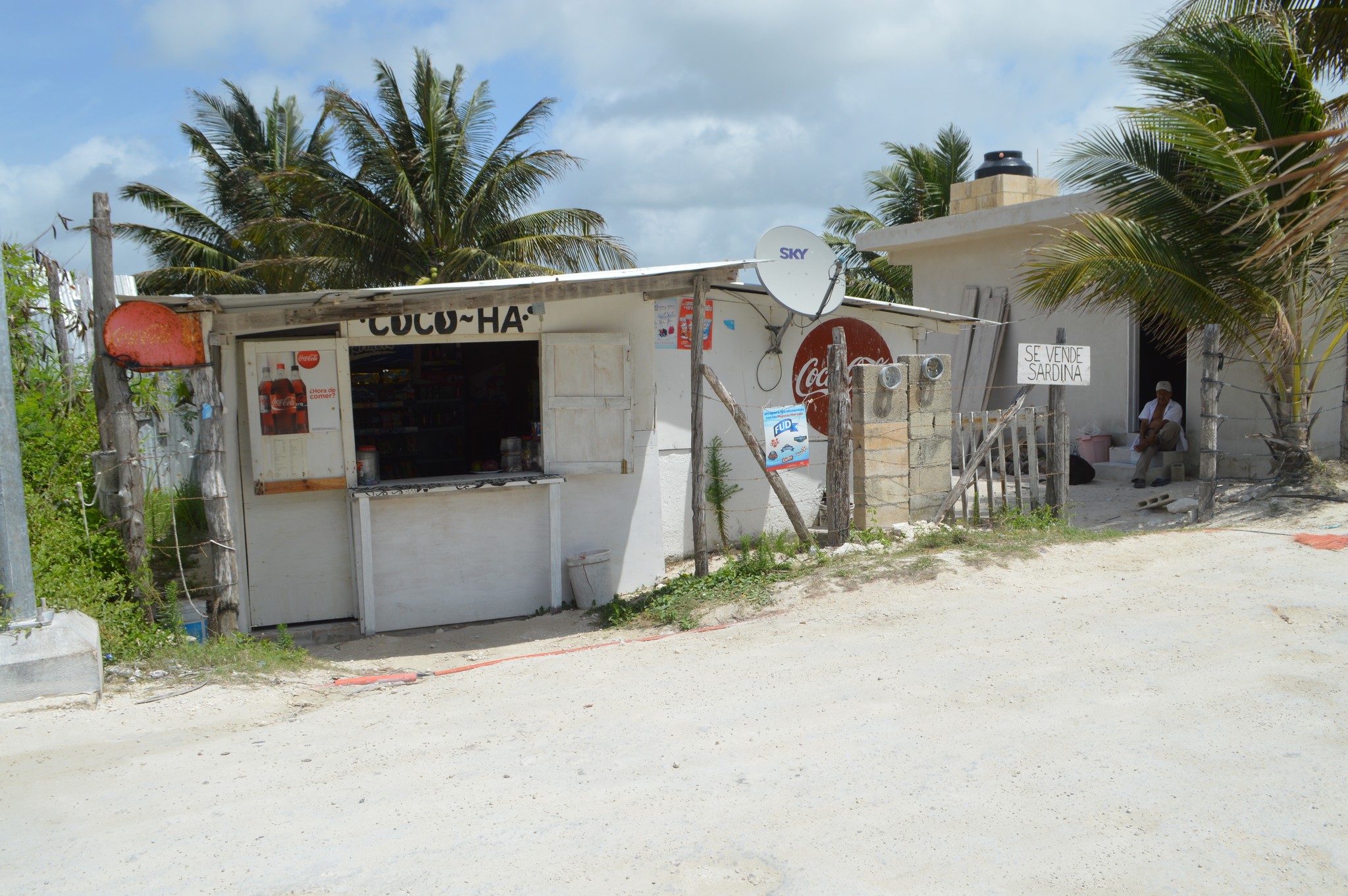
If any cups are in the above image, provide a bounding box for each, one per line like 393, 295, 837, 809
703, 318, 712, 340
685, 314, 693, 341
680, 317, 688, 339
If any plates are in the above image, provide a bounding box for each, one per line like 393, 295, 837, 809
470, 469, 504, 474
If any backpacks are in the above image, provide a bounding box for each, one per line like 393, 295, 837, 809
1068, 454, 1095, 485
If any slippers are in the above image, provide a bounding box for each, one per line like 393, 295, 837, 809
1133, 478, 1145, 488
1150, 478, 1172, 487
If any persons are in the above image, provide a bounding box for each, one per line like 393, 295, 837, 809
1132, 381, 1190, 488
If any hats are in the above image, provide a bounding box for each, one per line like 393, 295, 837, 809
1156, 381, 1172, 392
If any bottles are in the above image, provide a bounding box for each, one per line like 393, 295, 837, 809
531, 436, 542, 472
380, 411, 402, 428
428, 347, 433, 361
521, 436, 532, 471
258, 367, 274, 435
657, 307, 669, 340
270, 364, 297, 435
356, 446, 380, 486
667, 306, 676, 338
290, 365, 309, 433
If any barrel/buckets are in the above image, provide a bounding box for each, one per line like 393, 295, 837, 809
176, 600, 208, 644
565, 549, 614, 609
1076, 434, 1112, 464
819, 500, 855, 526
